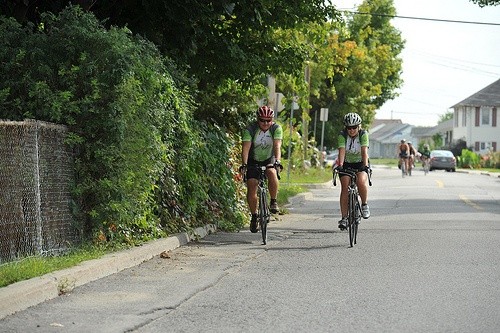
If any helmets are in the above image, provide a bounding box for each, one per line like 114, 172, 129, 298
400, 139, 406, 143
257, 106, 274, 119
343, 113, 362, 126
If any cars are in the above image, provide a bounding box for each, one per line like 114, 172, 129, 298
430, 149, 456, 172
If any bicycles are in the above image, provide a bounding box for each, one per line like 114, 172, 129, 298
332, 164, 372, 248
239, 163, 284, 246
398, 152, 431, 178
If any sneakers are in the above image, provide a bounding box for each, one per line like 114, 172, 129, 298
250, 217, 259, 233
270, 202, 279, 212
361, 203, 370, 219
338, 218, 348, 229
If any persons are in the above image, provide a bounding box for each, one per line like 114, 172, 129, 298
408, 142, 416, 172
423, 144, 431, 171
335, 113, 370, 229
242, 108, 283, 233
398, 139, 410, 175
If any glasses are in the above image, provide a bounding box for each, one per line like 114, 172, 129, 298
347, 125, 358, 130
259, 118, 271, 122
